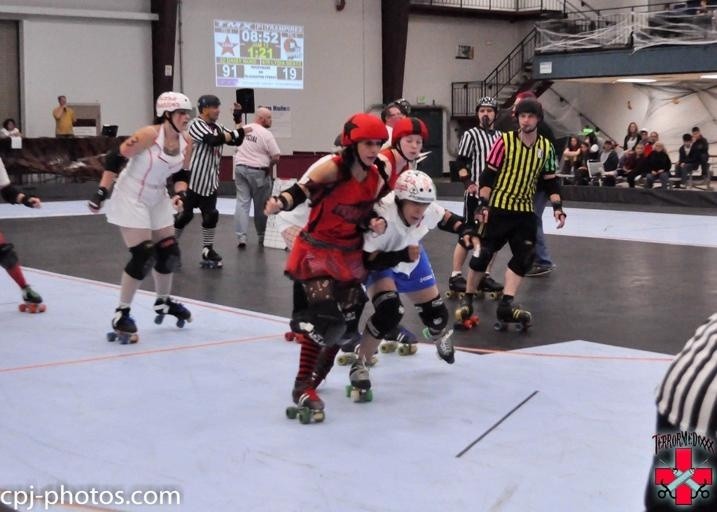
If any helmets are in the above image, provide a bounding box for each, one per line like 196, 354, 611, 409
340, 114, 389, 146
515, 98, 543, 122
156, 92, 193, 118
395, 99, 410, 115
197, 95, 220, 114
395, 170, 436, 205
391, 117, 428, 145
475, 97, 501, 118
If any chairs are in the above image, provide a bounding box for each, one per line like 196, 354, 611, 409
316, 152, 331, 156
293, 151, 314, 156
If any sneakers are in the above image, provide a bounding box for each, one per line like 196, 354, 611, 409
524, 263, 557, 276
153, 295, 192, 328
199, 245, 222, 269
237, 235, 247, 248
17, 288, 46, 313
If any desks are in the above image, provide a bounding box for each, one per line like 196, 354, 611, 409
0, 135, 129, 185
219, 156, 329, 182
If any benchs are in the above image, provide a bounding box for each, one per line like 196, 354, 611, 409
671, 170, 694, 188
557, 173, 606, 187
636, 177, 682, 190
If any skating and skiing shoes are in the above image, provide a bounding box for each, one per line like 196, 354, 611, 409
444, 272, 502, 332
493, 301, 531, 331
422, 328, 455, 363
338, 322, 417, 403
286, 381, 325, 424
107, 308, 140, 344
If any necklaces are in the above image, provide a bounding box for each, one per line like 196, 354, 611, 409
164, 133, 179, 148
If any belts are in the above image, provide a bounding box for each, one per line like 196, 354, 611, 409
238, 164, 265, 170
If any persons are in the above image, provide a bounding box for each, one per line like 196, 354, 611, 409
174, 94, 245, 261
0, 118, 22, 139
232, 106, 281, 249
101, 90, 194, 335
644, 313, 715, 510
260, 92, 716, 425
89, 135, 191, 214
52, 95, 77, 138
0, 157, 45, 305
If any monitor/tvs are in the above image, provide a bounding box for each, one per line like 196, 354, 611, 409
102, 125, 118, 136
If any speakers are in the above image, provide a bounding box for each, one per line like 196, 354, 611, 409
236, 88, 255, 114
